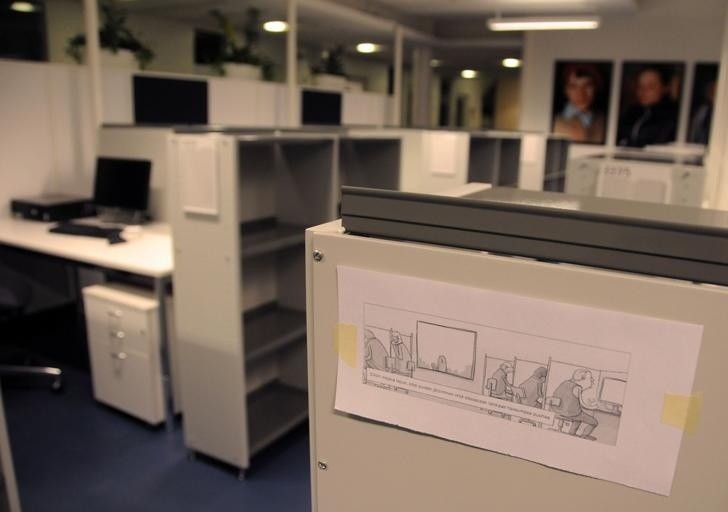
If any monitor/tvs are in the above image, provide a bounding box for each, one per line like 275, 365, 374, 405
301, 89, 342, 125
92, 157, 151, 213
131, 74, 210, 124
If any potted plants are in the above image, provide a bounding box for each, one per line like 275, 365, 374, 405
309, 41, 350, 93
208, 2, 264, 77
66, 1, 152, 74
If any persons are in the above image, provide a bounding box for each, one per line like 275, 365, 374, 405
690, 81, 714, 144
617, 64, 676, 146
551, 70, 604, 144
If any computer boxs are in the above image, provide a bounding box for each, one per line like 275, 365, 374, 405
11, 193, 98, 223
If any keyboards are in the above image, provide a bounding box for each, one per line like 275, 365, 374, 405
49, 223, 123, 238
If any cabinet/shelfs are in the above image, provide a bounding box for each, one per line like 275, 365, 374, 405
305, 83, 728, 512
82, 280, 170, 427
169, 125, 338, 471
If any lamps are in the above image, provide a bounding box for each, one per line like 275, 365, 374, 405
485, 0, 602, 33
